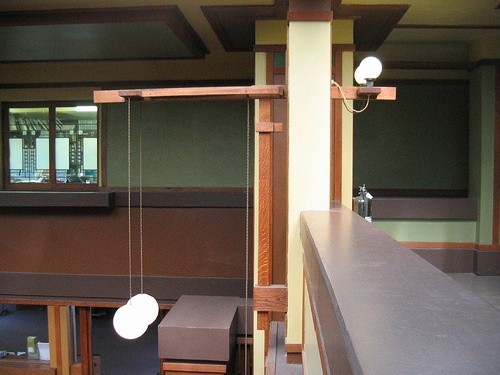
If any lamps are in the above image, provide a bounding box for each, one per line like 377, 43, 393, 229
130, 98, 160, 325
330, 56, 383, 114
112, 97, 150, 340
332, 65, 368, 113
